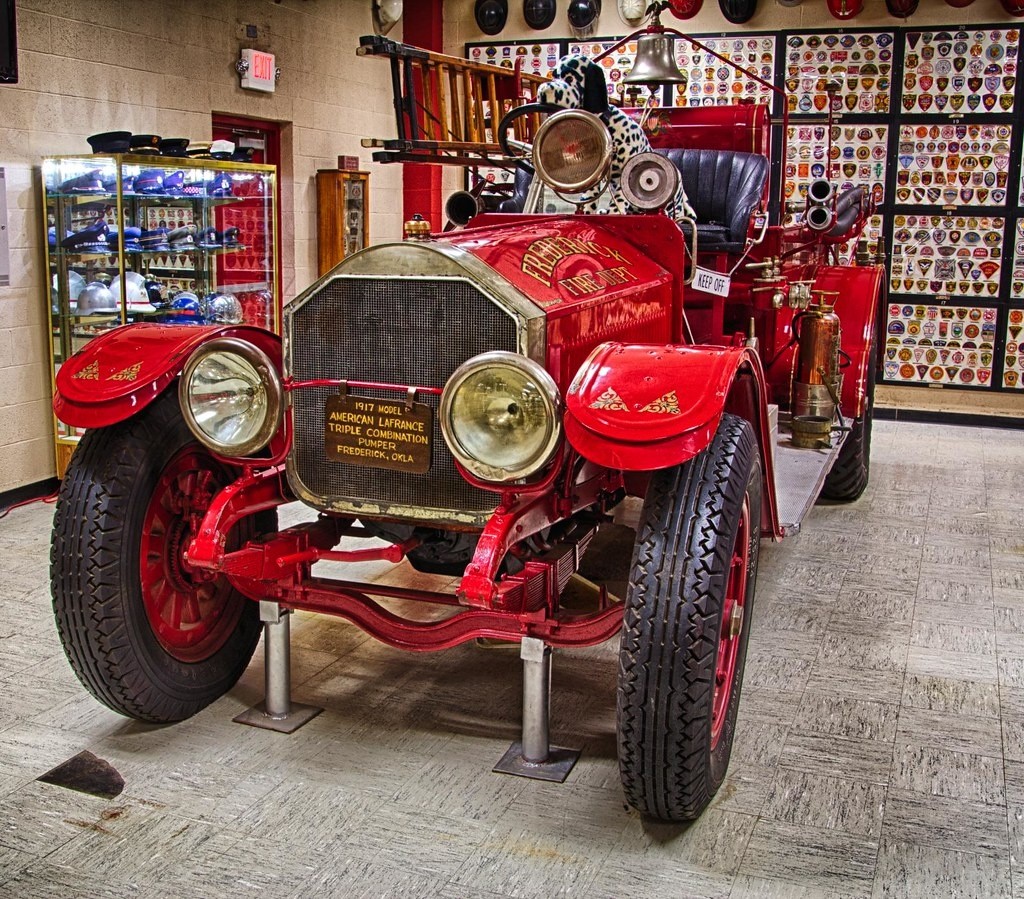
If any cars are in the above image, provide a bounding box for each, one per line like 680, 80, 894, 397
51, 3, 888, 824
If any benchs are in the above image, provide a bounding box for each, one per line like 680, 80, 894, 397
497, 148, 770, 252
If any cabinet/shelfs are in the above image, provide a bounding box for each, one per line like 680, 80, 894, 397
40, 153, 281, 478
317, 168, 370, 277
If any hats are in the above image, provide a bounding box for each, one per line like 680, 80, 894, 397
47, 219, 240, 254
87, 131, 255, 162
59, 169, 262, 197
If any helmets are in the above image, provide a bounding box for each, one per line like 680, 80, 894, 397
52, 271, 242, 325
372, 0, 1024, 44
